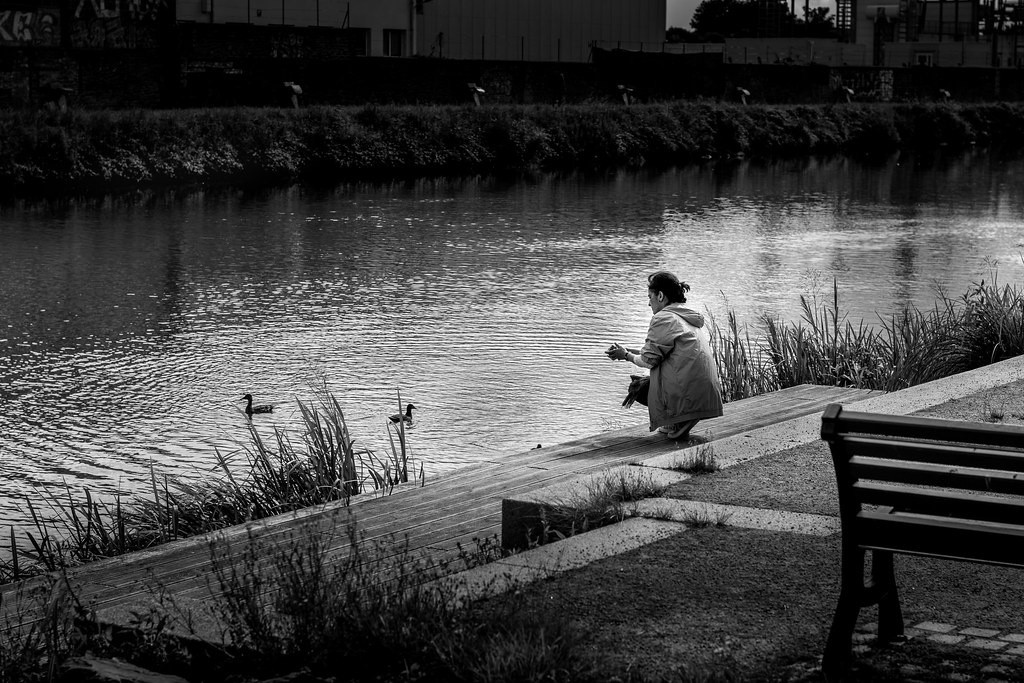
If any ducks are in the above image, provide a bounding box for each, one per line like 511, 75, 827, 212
240, 394, 273, 414
531, 444, 542, 450
388, 404, 417, 423
406, 417, 414, 426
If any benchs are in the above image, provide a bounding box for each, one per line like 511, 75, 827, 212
820, 405, 1024, 683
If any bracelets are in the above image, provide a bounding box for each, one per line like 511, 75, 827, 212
624, 352, 630, 362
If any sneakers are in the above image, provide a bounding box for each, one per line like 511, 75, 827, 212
658, 424, 675, 434
667, 420, 694, 439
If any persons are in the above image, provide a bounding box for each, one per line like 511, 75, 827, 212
605, 271, 724, 439
